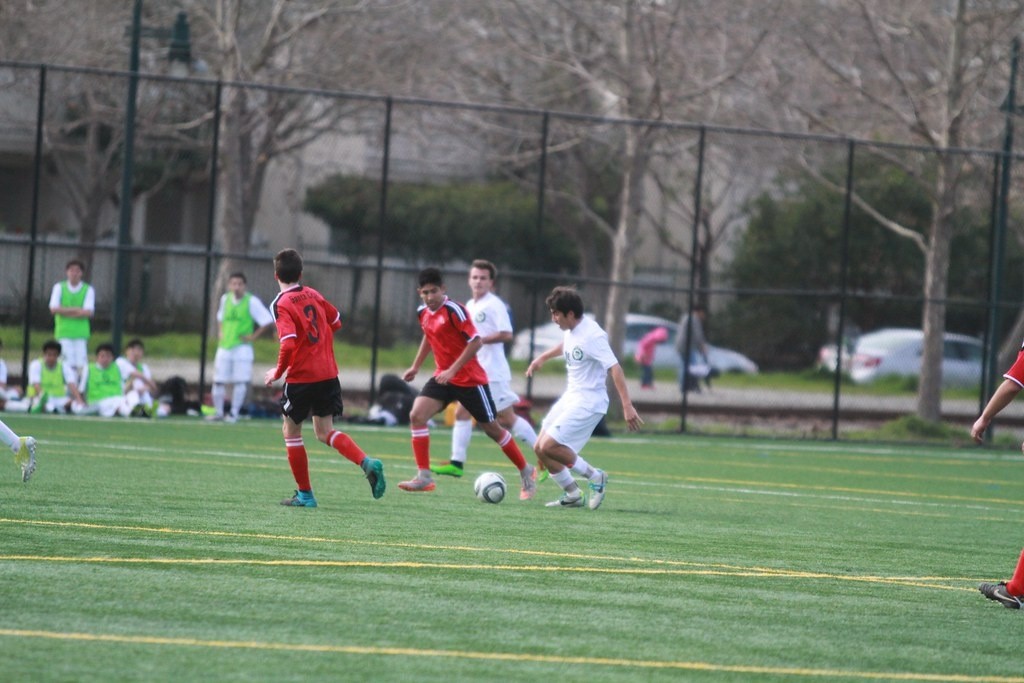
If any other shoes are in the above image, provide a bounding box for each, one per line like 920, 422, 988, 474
641, 385, 656, 392
224, 415, 236, 423
206, 415, 223, 422
281, 491, 317, 508
366, 460, 387, 500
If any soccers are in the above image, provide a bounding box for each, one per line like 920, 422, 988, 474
474, 471, 507, 504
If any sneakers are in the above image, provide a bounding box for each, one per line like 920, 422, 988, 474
537, 459, 548, 482
399, 476, 435, 491
16, 436, 36, 482
430, 463, 463, 478
519, 467, 537, 500
545, 491, 586, 508
589, 468, 610, 510
979, 581, 1024, 610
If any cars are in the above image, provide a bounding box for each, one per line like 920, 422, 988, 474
510, 312, 760, 379
848, 329, 986, 382
817, 340, 858, 378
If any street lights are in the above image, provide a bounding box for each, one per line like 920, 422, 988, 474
109, 0, 192, 359
978, 35, 1023, 445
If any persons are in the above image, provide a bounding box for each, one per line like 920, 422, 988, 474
971, 341, 1024, 609
49, 260, 94, 372
0, 420, 37, 479
525, 287, 644, 510
0, 339, 85, 415
637, 328, 668, 391
429, 259, 550, 481
205, 274, 274, 420
677, 305, 710, 394
398, 268, 537, 501
81, 341, 198, 419
266, 249, 386, 505
